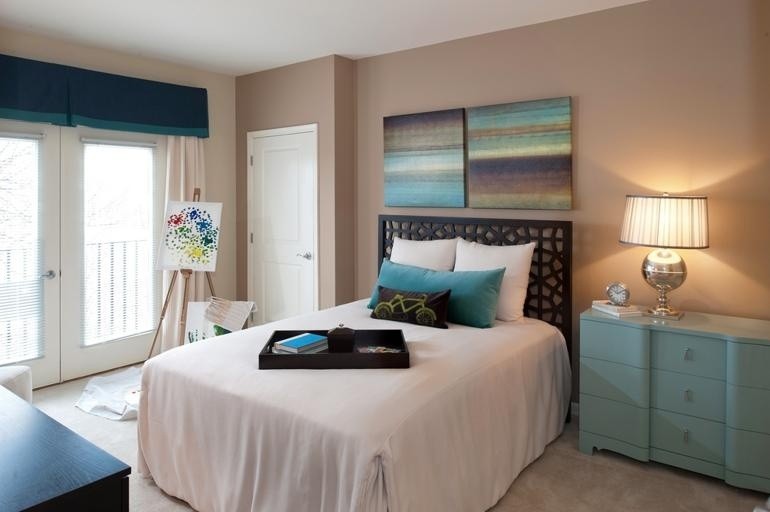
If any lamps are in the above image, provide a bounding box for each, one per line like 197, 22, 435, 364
618, 192, 710, 321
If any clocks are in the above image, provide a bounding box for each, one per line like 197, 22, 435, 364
604, 282, 630, 306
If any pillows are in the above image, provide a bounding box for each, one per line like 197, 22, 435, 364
366, 235, 538, 330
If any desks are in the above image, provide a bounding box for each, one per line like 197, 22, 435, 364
0, 384, 133, 511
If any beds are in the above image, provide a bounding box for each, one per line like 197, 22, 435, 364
140, 214, 574, 512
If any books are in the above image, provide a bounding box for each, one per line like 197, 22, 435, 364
591, 304, 643, 318
593, 299, 639, 312
271, 343, 328, 354
274, 332, 329, 353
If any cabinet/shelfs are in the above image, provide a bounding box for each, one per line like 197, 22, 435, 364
578, 306, 770, 497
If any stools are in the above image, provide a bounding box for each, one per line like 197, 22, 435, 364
0, 364, 33, 405
